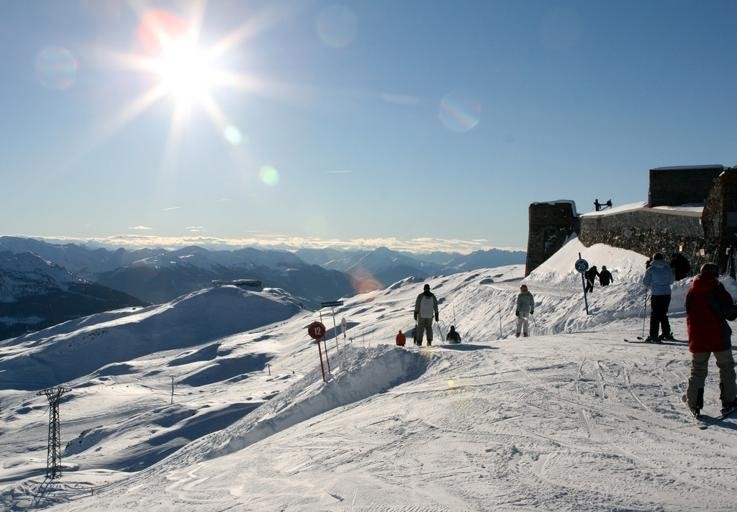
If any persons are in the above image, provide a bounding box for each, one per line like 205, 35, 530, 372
394, 329, 406, 346
514, 284, 534, 337
409, 324, 418, 344
584, 265, 599, 293
412, 284, 438, 347
641, 252, 674, 343
681, 262, 736, 417
444, 325, 461, 344
597, 265, 612, 286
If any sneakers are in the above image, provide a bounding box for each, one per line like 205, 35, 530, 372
646, 334, 671, 341
686, 401, 737, 414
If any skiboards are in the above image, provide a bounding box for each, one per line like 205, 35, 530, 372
625, 332, 688, 346
682, 394, 734, 430
